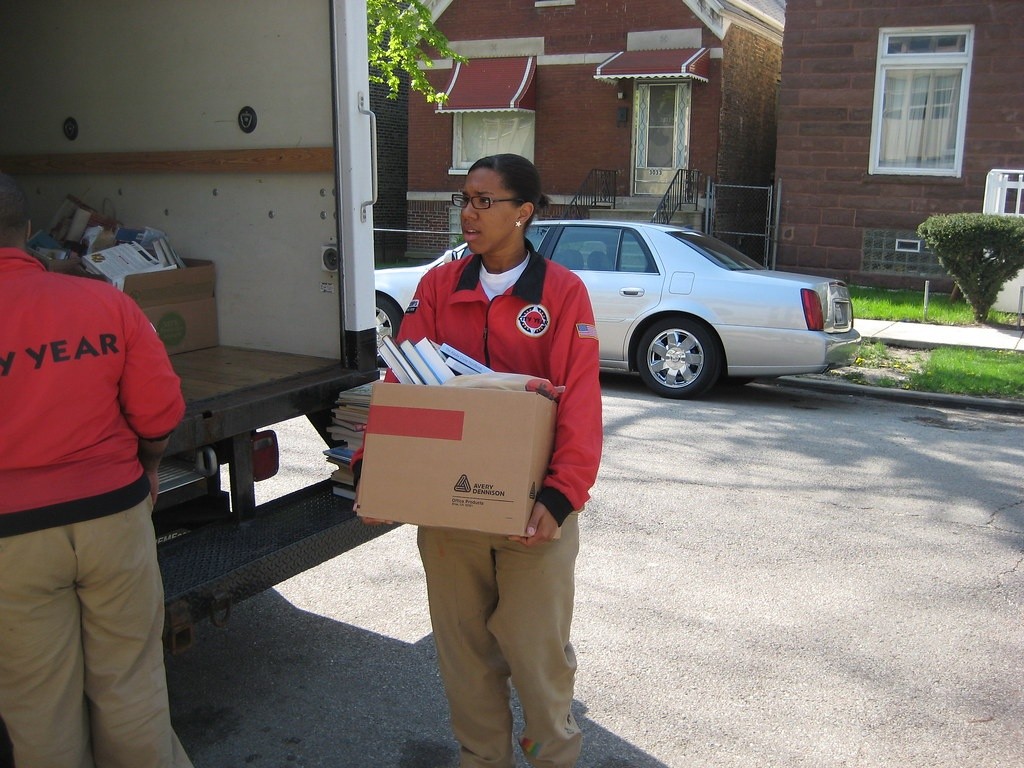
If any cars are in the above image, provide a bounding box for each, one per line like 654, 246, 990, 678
373, 220, 863, 400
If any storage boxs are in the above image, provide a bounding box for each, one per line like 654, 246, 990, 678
29, 247, 81, 277
68, 257, 220, 356
355, 382, 559, 536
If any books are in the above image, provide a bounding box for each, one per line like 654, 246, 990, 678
323, 380, 383, 499
377, 335, 495, 385
26, 194, 185, 292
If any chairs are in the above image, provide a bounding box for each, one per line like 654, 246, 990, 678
588, 251, 610, 271
559, 249, 583, 270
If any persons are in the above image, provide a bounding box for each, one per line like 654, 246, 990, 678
349, 153, 605, 768
0, 172, 186, 768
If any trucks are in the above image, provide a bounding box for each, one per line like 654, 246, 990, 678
1, 0, 416, 634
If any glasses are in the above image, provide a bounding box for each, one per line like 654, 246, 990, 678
451, 194, 525, 209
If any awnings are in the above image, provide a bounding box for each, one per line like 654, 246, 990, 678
433, 56, 537, 113
592, 47, 711, 86
